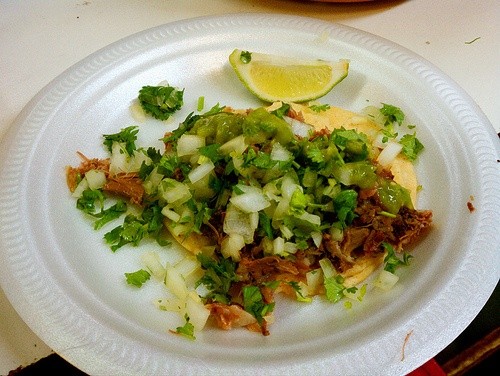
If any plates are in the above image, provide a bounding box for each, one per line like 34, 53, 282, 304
0, 12, 500, 376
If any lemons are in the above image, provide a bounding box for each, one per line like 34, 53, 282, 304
229, 49, 350, 104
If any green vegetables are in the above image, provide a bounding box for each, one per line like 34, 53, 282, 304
64, 84, 424, 338
